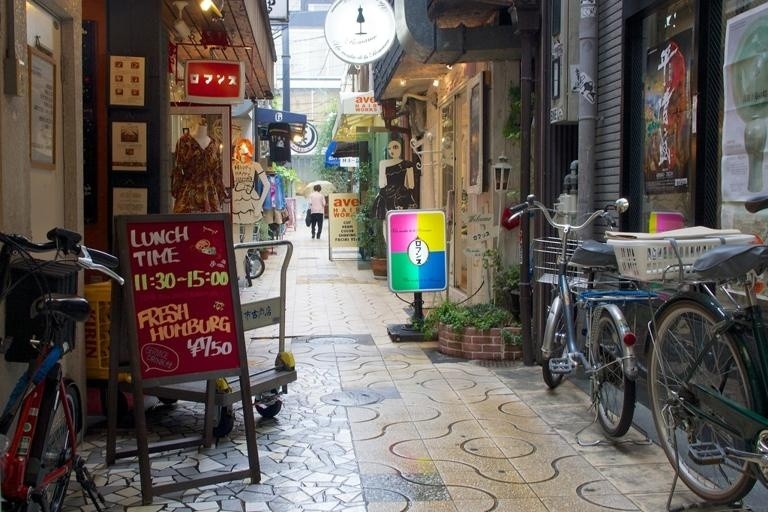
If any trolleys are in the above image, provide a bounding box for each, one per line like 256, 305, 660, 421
86, 237, 299, 435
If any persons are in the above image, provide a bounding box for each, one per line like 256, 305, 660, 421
309, 185, 326, 239
171, 119, 227, 214
230, 137, 271, 280
371, 134, 416, 243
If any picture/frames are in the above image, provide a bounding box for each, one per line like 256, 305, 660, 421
27, 44, 58, 171
106, 53, 152, 237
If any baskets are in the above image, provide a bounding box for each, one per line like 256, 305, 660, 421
606, 231, 755, 282
531, 235, 601, 288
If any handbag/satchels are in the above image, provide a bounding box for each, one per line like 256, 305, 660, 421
305, 208, 311, 226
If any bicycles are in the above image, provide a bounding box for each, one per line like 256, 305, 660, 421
0, 225, 127, 512
506, 193, 658, 448
605, 195, 768, 512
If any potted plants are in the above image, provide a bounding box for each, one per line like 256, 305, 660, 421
411, 301, 521, 362
353, 186, 387, 277
